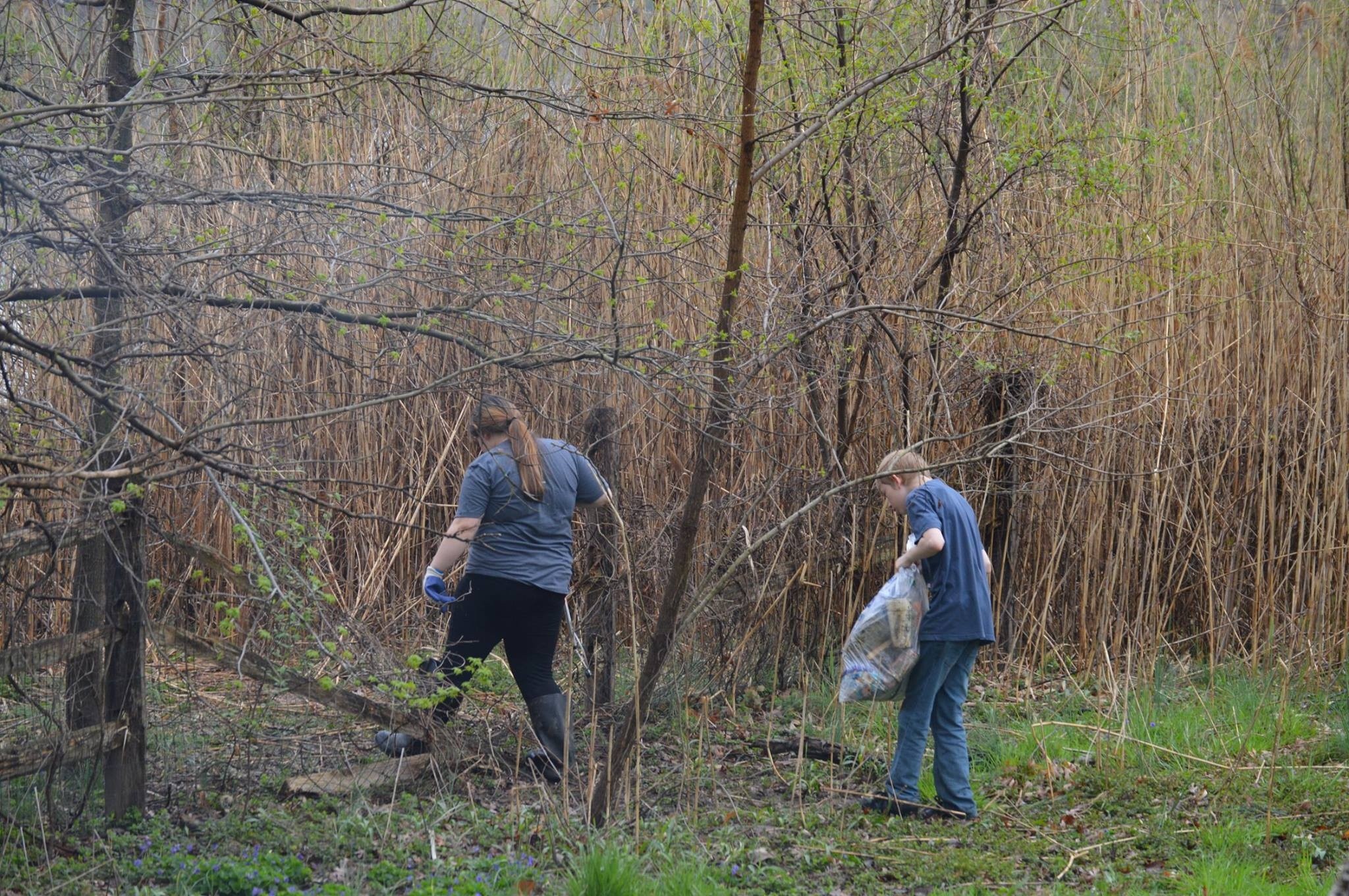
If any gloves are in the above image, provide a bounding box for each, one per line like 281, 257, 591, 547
423, 565, 456, 613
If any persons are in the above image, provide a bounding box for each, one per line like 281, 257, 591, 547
861, 450, 996, 820
376, 398, 613, 784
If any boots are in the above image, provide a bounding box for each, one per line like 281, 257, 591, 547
374, 698, 464, 754
527, 693, 572, 783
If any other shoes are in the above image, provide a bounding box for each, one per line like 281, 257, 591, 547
862, 792, 918, 818
923, 796, 967, 821
